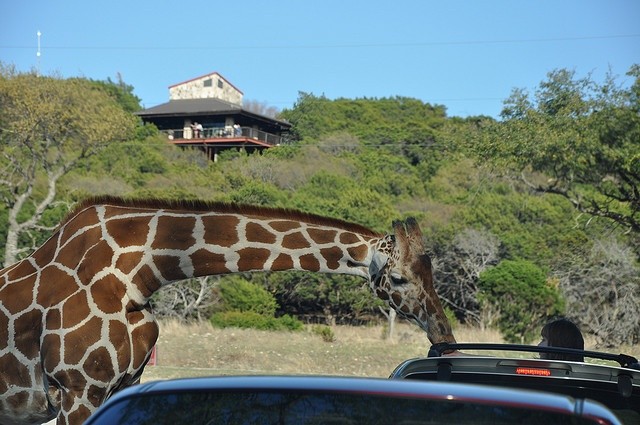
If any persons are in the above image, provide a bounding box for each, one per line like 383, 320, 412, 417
439, 321, 584, 364
234, 124, 237, 137
195, 124, 202, 137
237, 124, 242, 136
190, 122, 197, 138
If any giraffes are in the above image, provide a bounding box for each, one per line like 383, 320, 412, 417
0, 194, 456, 425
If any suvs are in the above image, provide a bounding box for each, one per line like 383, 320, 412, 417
387, 342, 640, 425
78, 375, 623, 425
